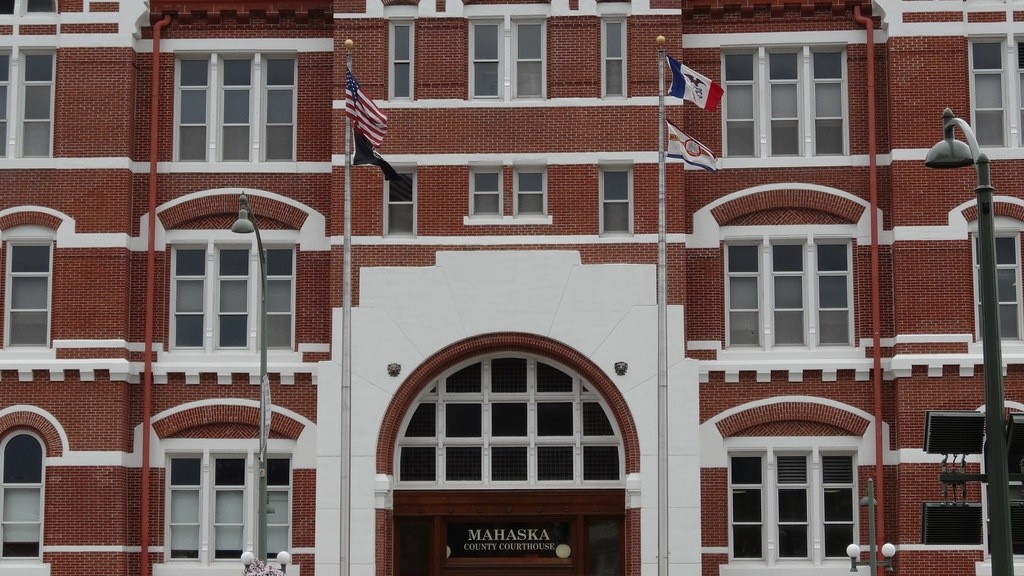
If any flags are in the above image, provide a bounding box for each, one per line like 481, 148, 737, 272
350, 125, 399, 183
666, 54, 724, 112
665, 119, 718, 173
343, 65, 389, 148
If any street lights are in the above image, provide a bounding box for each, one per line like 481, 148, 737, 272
846, 478, 894, 576
233, 187, 268, 567
921, 103, 1018, 576
